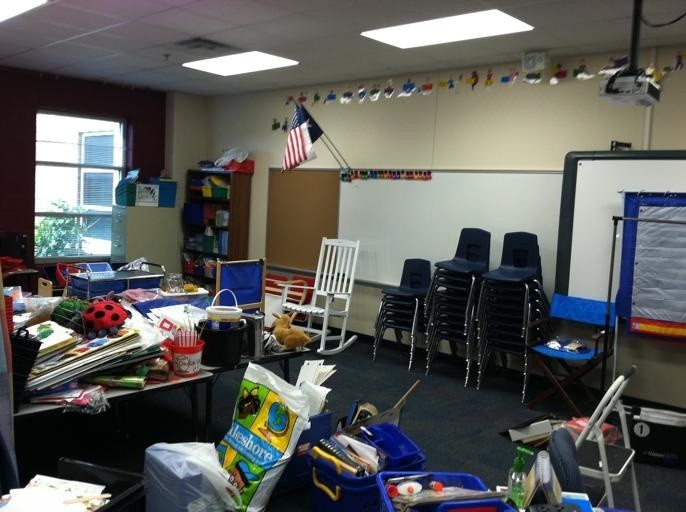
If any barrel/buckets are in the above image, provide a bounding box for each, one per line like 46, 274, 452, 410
207, 289, 241, 329
197, 318, 245, 368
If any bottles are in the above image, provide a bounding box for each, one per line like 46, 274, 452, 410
164, 273, 184, 293
396, 480, 423, 495
246, 309, 266, 362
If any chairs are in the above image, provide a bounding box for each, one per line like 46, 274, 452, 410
36, 278, 53, 297
530, 359, 645, 512
536, 292, 621, 412
276, 231, 360, 357
370, 227, 554, 404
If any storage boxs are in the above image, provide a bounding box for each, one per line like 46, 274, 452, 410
302, 412, 429, 512
111, 180, 179, 206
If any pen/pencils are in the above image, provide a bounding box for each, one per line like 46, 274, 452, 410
173, 327, 198, 347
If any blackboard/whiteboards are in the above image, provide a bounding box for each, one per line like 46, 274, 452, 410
266, 169, 565, 296
566, 156, 685, 303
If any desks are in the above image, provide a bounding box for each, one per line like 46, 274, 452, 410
1, 288, 214, 454
132, 295, 313, 446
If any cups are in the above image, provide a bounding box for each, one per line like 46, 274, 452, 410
169, 339, 205, 377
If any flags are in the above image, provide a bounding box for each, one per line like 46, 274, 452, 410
278, 104, 323, 174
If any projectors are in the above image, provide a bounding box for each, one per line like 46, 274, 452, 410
598, 76, 660, 108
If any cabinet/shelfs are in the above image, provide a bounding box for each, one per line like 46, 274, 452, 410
180, 159, 256, 297
111, 206, 180, 276
65, 262, 165, 299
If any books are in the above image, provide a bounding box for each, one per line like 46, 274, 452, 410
0, 471, 111, 512
522, 450, 562, 509
313, 432, 380, 479
15, 298, 210, 398
184, 210, 228, 252
398, 482, 504, 509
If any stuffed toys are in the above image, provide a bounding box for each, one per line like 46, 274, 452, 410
271, 309, 311, 353
